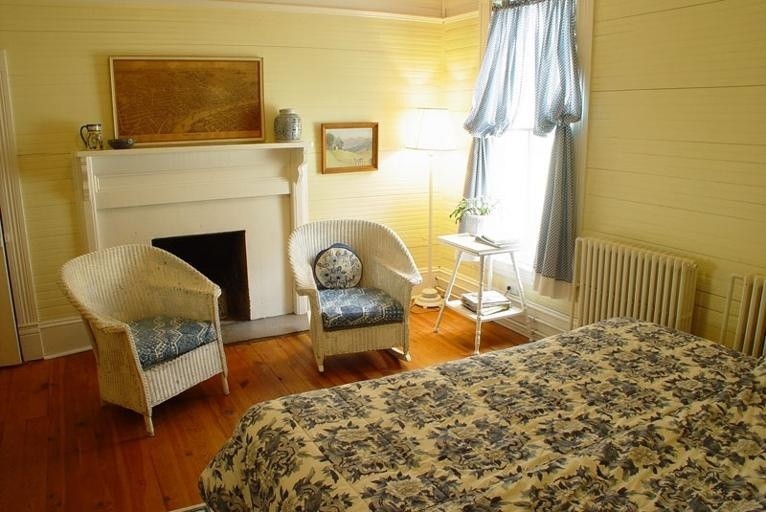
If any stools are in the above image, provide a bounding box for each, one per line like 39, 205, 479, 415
426, 227, 536, 357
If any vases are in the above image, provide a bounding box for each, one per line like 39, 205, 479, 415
272, 105, 304, 142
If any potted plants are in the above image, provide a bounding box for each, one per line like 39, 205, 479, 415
446, 192, 506, 239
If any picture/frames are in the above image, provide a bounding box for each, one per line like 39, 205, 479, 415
318, 121, 380, 176
102, 50, 272, 153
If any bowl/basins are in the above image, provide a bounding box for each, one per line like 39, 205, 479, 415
107, 137, 136, 149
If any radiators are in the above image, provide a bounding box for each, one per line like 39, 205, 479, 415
717, 266, 766, 359
559, 233, 702, 342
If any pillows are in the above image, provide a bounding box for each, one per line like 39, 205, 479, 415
311, 240, 363, 291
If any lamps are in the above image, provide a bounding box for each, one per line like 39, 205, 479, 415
397, 106, 470, 309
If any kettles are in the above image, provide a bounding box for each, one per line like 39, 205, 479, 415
80, 123, 105, 151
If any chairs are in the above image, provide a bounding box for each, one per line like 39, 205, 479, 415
283, 217, 423, 376
53, 240, 231, 437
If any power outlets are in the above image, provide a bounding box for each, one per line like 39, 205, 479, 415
504, 281, 517, 294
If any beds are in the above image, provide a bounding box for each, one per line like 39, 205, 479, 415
194, 306, 766, 512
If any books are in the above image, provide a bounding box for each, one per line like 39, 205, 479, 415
460, 289, 511, 316
474, 235, 517, 249
481, 235, 510, 242
